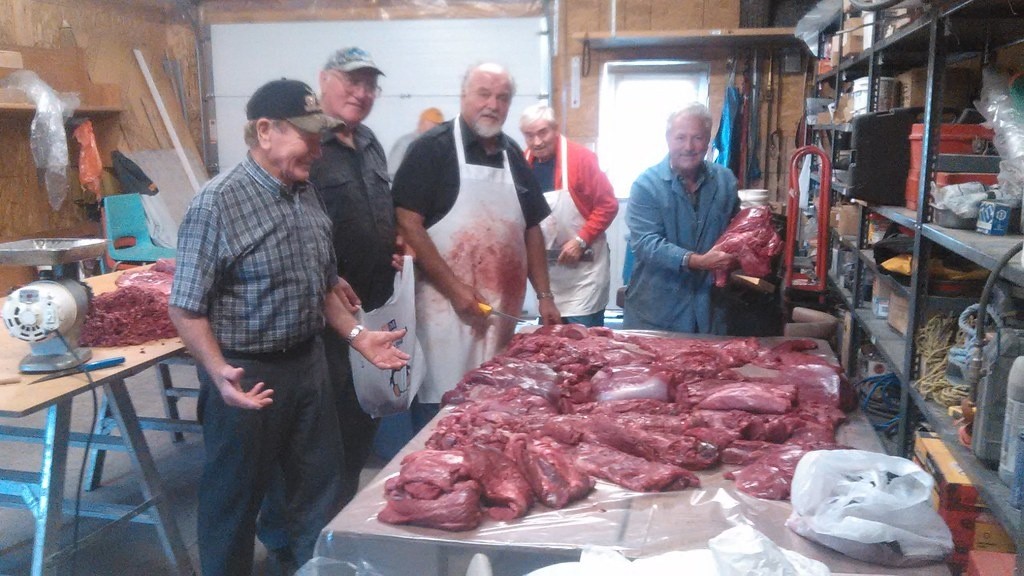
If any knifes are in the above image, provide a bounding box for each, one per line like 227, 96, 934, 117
478, 302, 526, 325
27, 357, 125, 385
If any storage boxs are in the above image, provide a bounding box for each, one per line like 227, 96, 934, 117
814, 1, 1001, 334
911, 428, 1017, 575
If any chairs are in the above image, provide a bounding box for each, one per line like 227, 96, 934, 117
103, 193, 176, 271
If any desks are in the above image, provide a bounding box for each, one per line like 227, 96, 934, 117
0, 266, 224, 576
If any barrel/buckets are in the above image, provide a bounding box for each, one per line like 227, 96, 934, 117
854, 76, 899, 116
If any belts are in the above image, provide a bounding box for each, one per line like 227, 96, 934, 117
223, 335, 315, 361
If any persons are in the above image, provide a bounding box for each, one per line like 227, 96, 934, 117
518, 104, 619, 328
317, 47, 417, 503
391, 59, 563, 437
167, 78, 412, 576
622, 101, 741, 336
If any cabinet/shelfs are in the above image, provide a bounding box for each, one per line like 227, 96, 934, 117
760, 1, 1024, 576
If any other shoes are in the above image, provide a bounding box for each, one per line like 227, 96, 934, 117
257, 525, 298, 575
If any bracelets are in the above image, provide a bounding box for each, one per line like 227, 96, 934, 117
537, 292, 554, 299
345, 324, 365, 345
390, 363, 411, 397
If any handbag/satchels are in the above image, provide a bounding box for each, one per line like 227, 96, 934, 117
786, 448, 953, 566
349, 254, 427, 419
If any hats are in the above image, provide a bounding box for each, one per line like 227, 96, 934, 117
325, 46, 386, 78
247, 78, 346, 133
421, 109, 443, 124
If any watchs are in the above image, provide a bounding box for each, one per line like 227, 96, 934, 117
576, 237, 587, 250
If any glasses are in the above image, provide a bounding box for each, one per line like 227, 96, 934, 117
330, 71, 383, 98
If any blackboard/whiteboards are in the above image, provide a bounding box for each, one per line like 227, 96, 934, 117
210, 15, 553, 174
